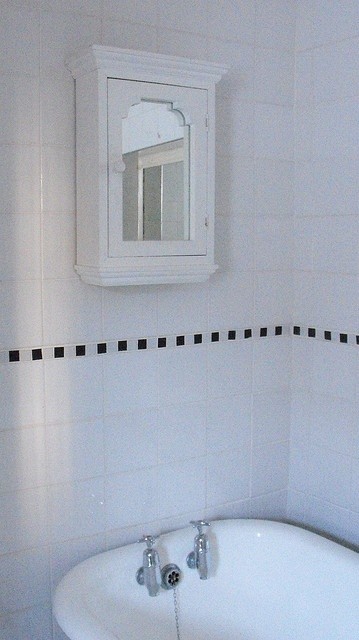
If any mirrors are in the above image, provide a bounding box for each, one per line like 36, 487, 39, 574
121, 98, 192, 243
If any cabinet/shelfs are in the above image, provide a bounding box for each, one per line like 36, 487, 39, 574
64, 43, 231, 288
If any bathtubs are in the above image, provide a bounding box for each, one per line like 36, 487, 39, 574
51, 519, 358, 640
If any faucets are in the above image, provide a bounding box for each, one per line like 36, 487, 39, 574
187, 520, 211, 581
137, 533, 164, 596
